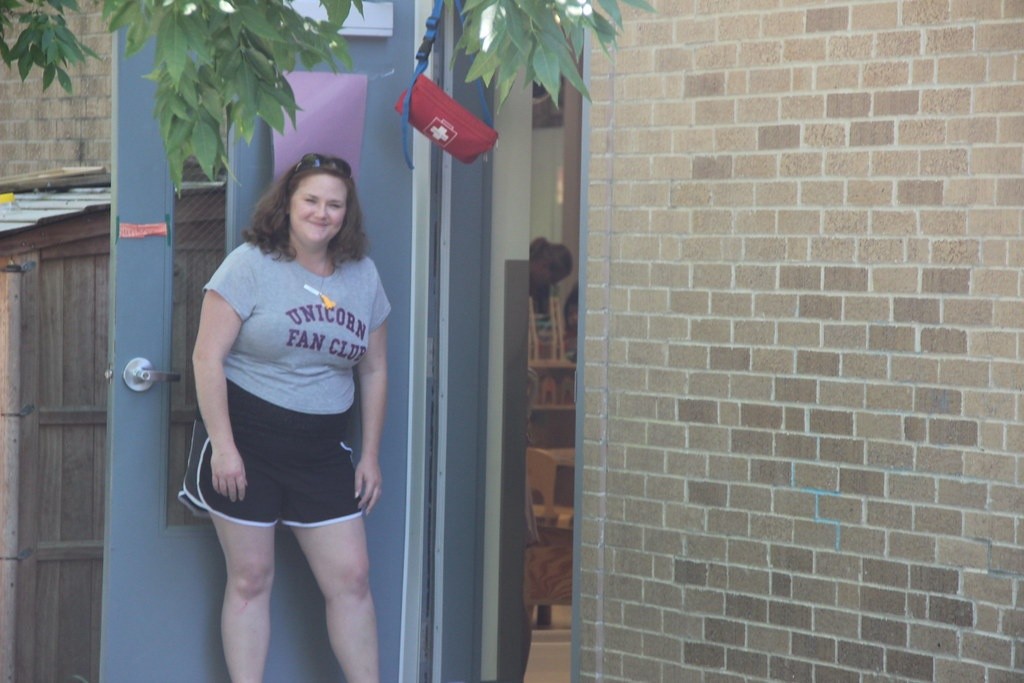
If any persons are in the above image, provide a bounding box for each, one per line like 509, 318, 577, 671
178, 153, 394, 683
529, 236, 574, 314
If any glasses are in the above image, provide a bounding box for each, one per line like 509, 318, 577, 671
295, 153, 352, 179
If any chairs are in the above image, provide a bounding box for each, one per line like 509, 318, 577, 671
526, 281, 579, 626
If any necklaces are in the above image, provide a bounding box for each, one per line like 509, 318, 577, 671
295, 256, 337, 310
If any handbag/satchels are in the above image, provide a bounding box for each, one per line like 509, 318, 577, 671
395, 0, 499, 166
